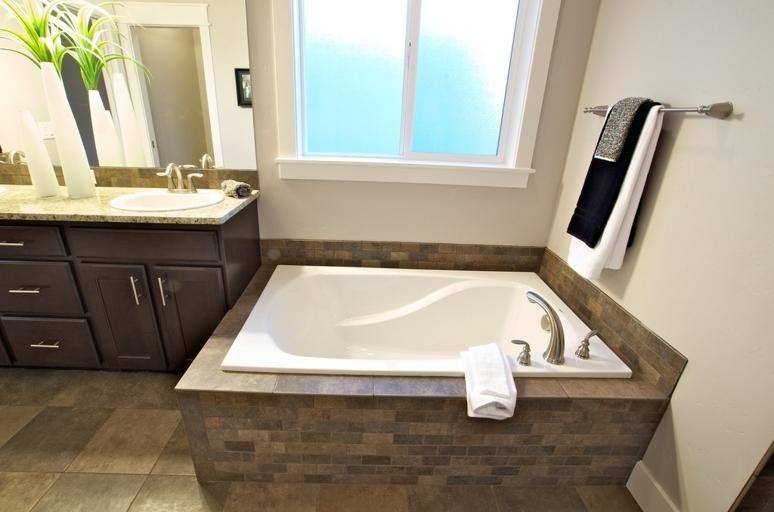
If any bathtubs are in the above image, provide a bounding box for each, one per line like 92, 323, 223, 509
221, 264, 633, 378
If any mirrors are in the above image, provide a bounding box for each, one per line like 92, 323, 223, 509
0, 1, 258, 173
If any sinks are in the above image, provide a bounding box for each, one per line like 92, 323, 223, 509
108, 187, 224, 213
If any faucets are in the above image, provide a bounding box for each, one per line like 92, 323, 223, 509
156, 163, 184, 189
6, 149, 26, 164
525, 290, 564, 365
201, 154, 213, 169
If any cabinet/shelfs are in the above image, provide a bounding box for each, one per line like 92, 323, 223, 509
63, 226, 227, 371
0, 225, 102, 370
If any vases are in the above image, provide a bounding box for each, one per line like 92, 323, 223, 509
16, 107, 57, 199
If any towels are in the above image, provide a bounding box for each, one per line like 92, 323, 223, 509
220, 178, 252, 200
463, 341, 518, 421
565, 96, 665, 283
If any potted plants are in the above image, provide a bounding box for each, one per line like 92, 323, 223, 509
44, 2, 153, 168
0, 1, 127, 200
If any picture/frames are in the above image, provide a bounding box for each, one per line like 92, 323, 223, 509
233, 68, 252, 107
